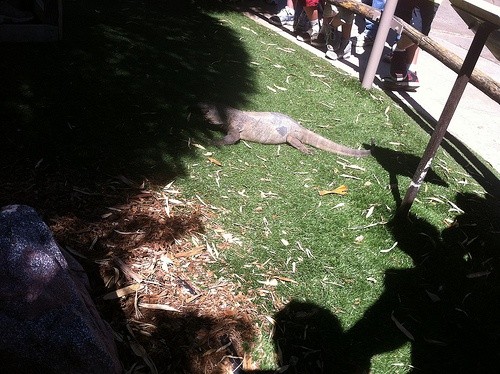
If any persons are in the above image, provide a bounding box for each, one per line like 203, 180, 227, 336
268, 0, 440, 91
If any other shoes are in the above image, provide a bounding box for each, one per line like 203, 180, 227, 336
296, 28, 320, 42
381, 69, 420, 90
269, 6, 297, 26
326, 41, 352, 60
